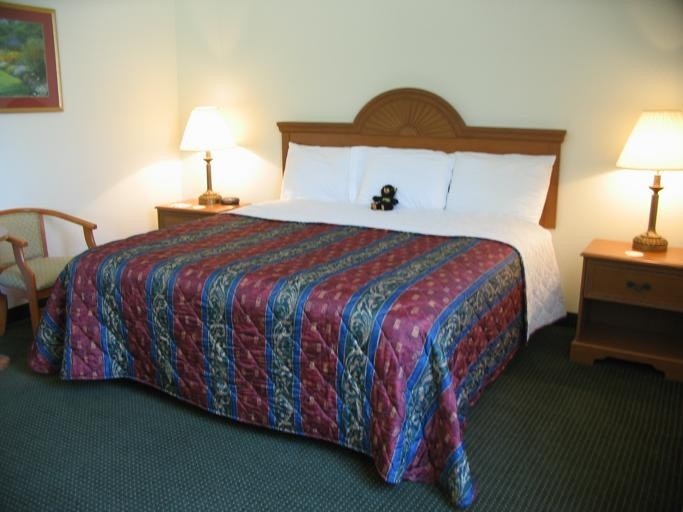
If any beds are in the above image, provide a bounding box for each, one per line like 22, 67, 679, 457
58, 86, 569, 484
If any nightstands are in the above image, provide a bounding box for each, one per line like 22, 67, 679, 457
155, 195, 251, 229
569, 237, 682, 384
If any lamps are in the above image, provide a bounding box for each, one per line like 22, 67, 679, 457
179, 106, 234, 207
614, 108, 683, 253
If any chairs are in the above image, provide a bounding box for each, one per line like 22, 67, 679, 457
0, 207, 102, 338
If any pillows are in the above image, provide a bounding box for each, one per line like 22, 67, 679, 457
278, 143, 557, 229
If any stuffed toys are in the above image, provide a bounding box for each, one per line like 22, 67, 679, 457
371, 184, 398, 211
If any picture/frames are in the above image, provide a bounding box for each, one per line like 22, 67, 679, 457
0, 3, 65, 115
